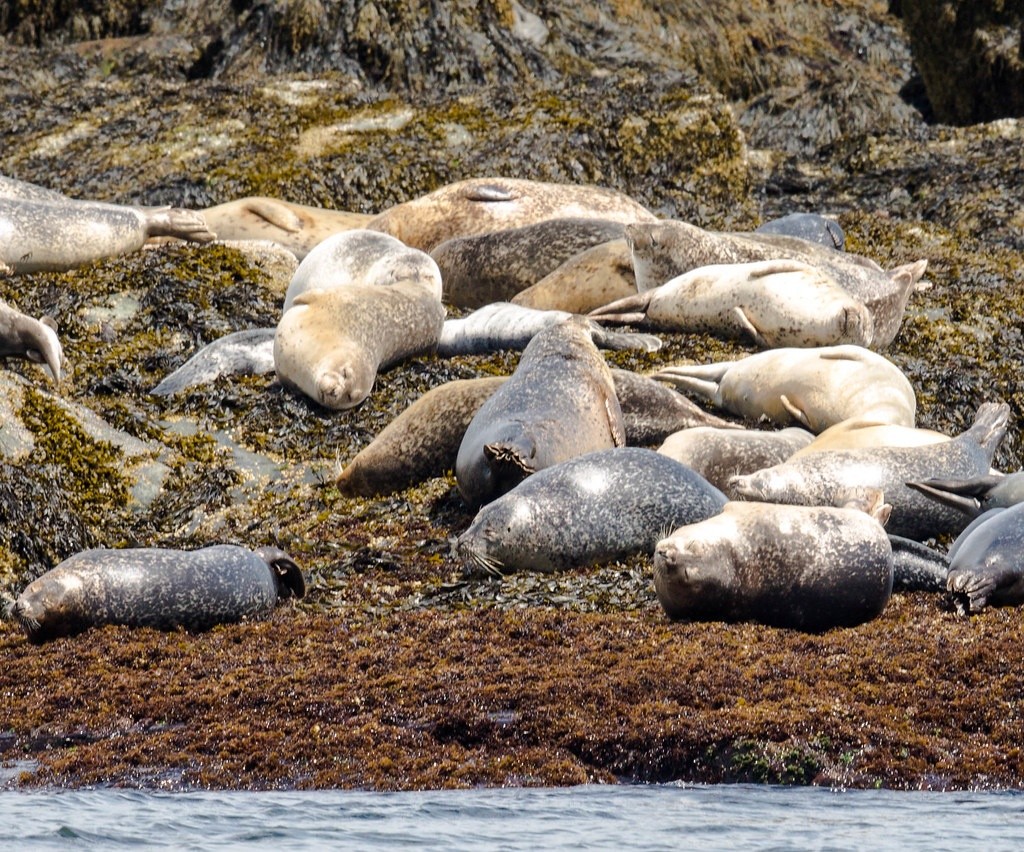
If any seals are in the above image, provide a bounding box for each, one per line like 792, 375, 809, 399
0, 171, 1024, 645
